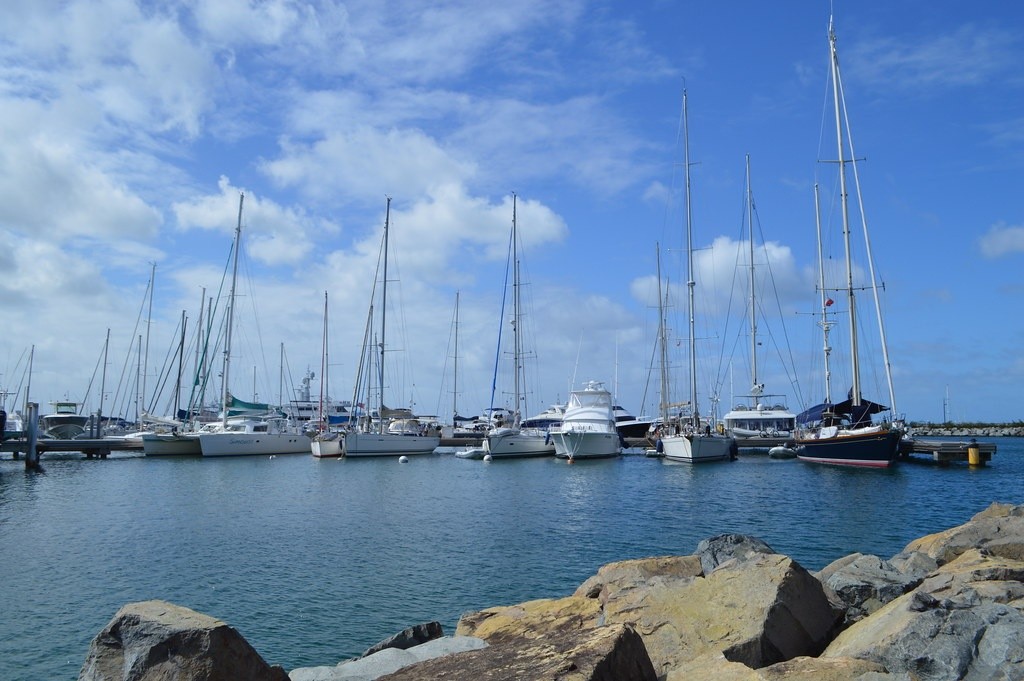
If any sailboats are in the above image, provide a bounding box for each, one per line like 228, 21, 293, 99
0, 16, 916, 468
